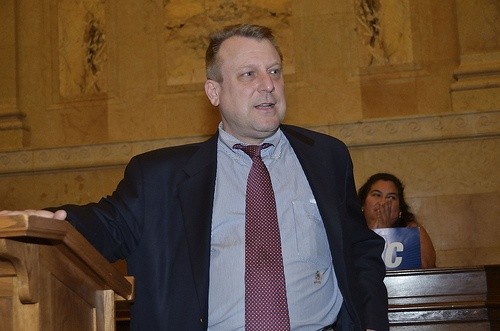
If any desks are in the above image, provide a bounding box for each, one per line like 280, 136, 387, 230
383, 265, 500, 331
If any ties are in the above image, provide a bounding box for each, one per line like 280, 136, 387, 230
232, 142, 290, 329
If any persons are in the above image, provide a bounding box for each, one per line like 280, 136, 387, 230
358, 173, 437, 268
0, 24, 389, 331
